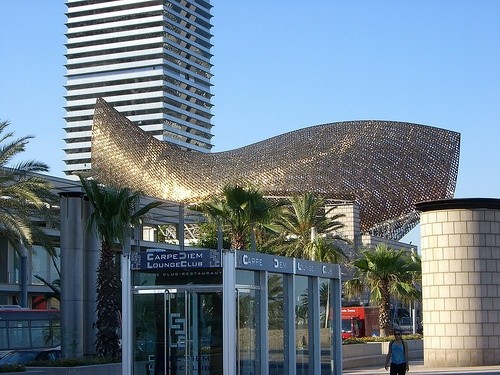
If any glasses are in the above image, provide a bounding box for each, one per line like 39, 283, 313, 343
394, 333, 401, 336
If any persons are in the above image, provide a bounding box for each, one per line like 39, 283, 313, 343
384, 329, 410, 375
